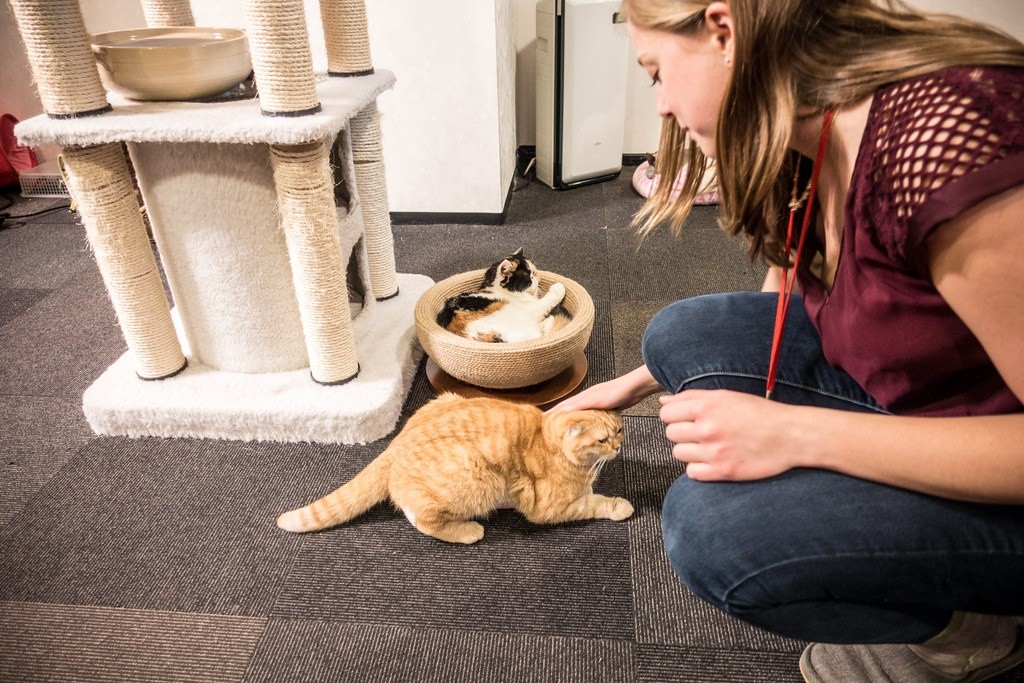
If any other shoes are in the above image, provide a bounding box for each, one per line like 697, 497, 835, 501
799, 617, 1024, 683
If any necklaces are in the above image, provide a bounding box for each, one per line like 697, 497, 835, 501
785, 109, 835, 211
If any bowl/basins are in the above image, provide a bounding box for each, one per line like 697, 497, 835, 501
89, 25, 253, 100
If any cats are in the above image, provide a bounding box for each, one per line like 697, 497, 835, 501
435, 245, 571, 344
645, 149, 718, 193
275, 388, 635, 545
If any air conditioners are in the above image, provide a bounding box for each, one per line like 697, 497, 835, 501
535, 0, 628, 189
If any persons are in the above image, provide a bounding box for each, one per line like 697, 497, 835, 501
544, 1, 1023, 683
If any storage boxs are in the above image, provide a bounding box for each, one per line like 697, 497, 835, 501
20, 161, 72, 199
